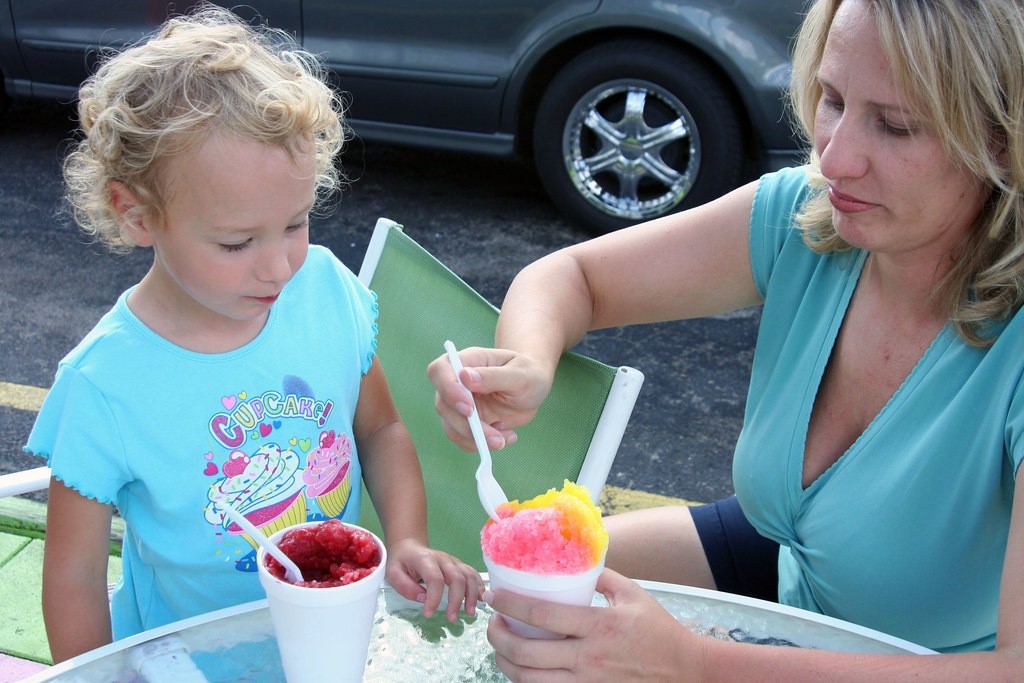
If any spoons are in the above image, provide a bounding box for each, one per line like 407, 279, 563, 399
443, 339, 509, 524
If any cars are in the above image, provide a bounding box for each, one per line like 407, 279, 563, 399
0, 0, 825, 237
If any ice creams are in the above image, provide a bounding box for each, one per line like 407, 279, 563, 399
480, 477, 610, 577
264, 518, 382, 588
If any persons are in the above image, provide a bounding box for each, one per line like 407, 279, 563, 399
22, 0, 485, 683
426, 0, 1024, 683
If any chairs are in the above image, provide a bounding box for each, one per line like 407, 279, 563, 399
353, 219, 644, 572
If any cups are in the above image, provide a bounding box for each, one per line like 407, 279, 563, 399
483, 551, 606, 641
256, 521, 387, 683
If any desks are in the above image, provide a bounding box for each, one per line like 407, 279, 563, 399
18, 572, 944, 683
